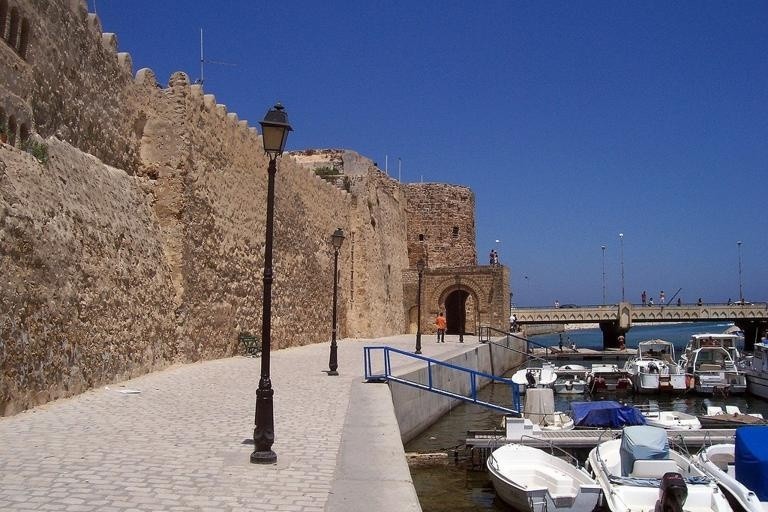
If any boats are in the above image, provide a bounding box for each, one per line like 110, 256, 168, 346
511, 333, 768, 399
691, 424, 767, 512
499, 387, 575, 431
566, 399, 647, 429
486, 432, 602, 512
695, 398, 767, 429
585, 424, 735, 512
632, 402, 702, 430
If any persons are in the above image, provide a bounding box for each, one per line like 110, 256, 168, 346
697, 298, 704, 306
740, 298, 745, 305
642, 291, 647, 307
617, 333, 624, 350
490, 249, 498, 264
558, 333, 563, 352
659, 291, 665, 304
435, 312, 446, 343
567, 337, 577, 353
510, 314, 522, 333
728, 298, 734, 306
676, 298, 681, 306
648, 297, 654, 307
554, 299, 560, 308
510, 304, 517, 309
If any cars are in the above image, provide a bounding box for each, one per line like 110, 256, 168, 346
559, 304, 580, 308
729, 301, 755, 306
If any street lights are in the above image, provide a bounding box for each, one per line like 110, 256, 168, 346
600, 244, 607, 307
251, 99, 293, 464
455, 273, 467, 343
736, 239, 744, 303
328, 228, 349, 379
619, 233, 627, 304
414, 256, 427, 356
